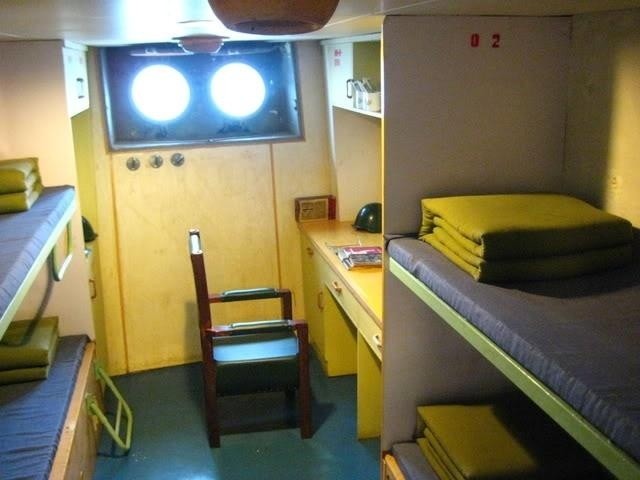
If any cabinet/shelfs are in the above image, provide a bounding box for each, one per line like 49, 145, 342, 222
0, 36, 110, 399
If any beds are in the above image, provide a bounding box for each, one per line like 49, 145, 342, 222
388, 235, 640, 479
0, 184, 78, 346
380, 441, 442, 480
1, 334, 107, 480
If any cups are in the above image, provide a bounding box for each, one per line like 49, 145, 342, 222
352, 89, 381, 114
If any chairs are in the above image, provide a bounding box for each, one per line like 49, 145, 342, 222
188, 227, 312, 449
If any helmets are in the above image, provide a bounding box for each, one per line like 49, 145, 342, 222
82, 216, 97, 242
352, 203, 381, 233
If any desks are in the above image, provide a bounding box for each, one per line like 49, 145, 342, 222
296, 219, 384, 453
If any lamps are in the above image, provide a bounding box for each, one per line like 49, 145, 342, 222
208, 0, 339, 35
172, 36, 229, 54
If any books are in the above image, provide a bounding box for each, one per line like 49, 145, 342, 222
336, 247, 382, 271
